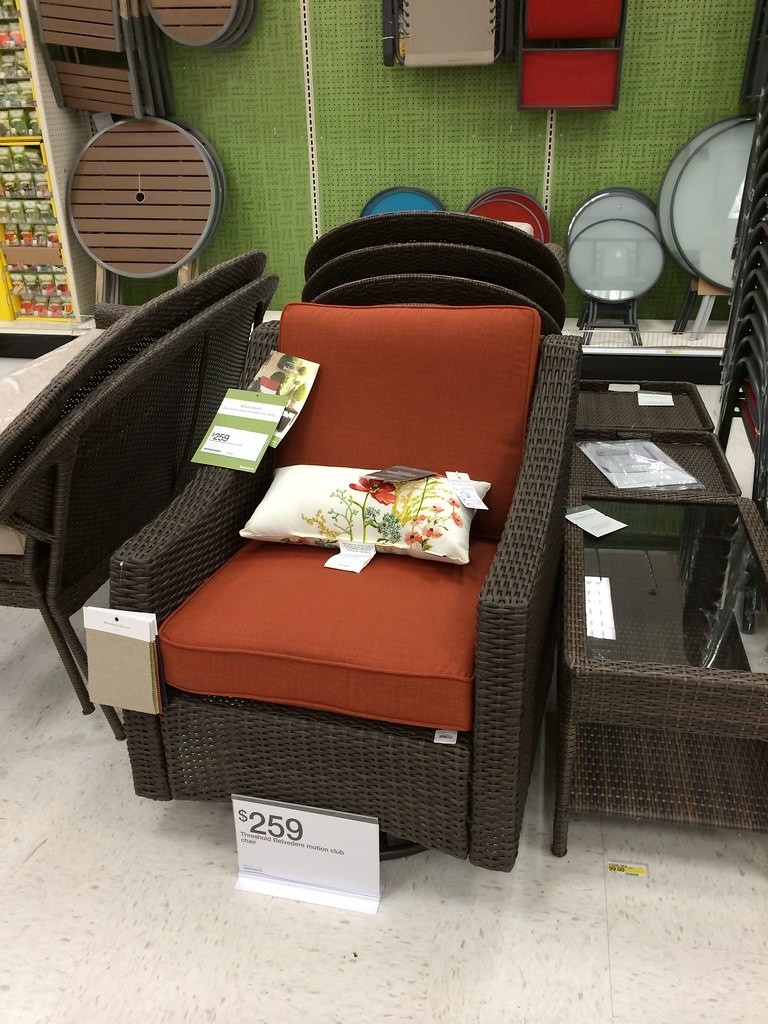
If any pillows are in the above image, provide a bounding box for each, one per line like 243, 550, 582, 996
239, 464, 491, 565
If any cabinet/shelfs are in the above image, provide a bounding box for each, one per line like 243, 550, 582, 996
0, 0, 96, 336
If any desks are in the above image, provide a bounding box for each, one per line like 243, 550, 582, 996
550, 487, 768, 857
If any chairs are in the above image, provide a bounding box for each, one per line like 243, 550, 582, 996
716, 88, 768, 522
301, 211, 567, 339
0, 249, 280, 741
34, 0, 173, 119
382, 0, 628, 112
107, 302, 581, 872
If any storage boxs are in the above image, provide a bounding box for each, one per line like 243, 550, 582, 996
576, 380, 715, 431
568, 428, 742, 499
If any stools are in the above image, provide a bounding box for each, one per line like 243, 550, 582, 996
64, 115, 222, 308
360, 186, 550, 247
146, 0, 260, 52
564, 115, 757, 347
